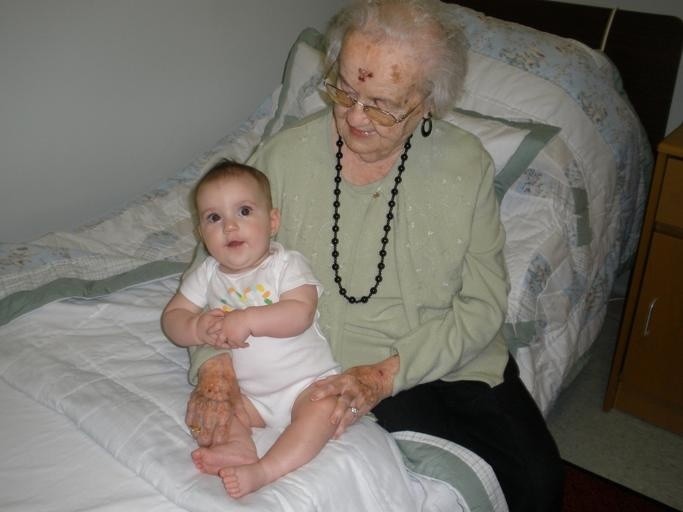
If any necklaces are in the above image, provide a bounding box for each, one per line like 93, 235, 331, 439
331, 134, 414, 304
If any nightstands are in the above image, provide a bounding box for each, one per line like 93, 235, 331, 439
601, 123, 682, 438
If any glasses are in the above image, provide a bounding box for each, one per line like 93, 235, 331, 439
323, 59, 429, 127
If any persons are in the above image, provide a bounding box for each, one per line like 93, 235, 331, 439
177, 0, 569, 512
161, 158, 347, 500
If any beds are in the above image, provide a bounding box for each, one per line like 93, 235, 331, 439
1, 2, 682, 512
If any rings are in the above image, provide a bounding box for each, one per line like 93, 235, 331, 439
350, 406, 360, 415
